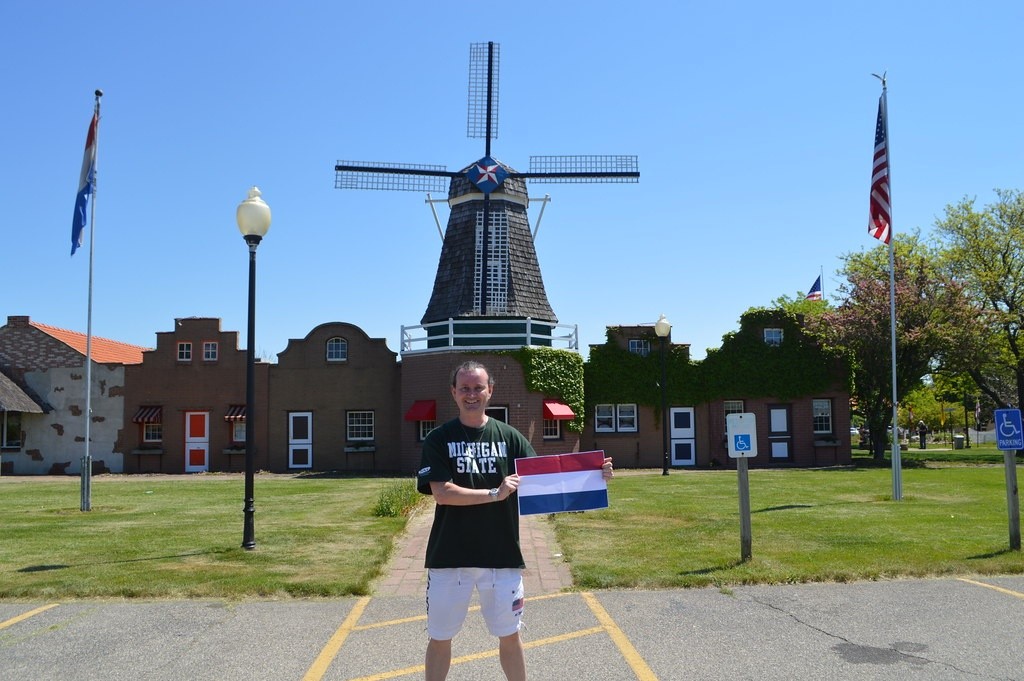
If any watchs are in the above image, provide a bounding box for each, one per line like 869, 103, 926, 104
489, 487, 499, 501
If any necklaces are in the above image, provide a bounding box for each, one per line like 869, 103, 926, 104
462, 425, 484, 460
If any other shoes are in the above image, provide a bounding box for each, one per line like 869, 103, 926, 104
919, 448, 927, 449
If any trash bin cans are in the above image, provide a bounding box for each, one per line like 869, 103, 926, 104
954, 435, 964, 449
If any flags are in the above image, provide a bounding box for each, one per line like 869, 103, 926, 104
515, 449, 610, 515
71, 105, 98, 256
908, 402, 914, 424
974, 400, 981, 425
940, 401, 946, 426
867, 93, 892, 246
802, 274, 822, 301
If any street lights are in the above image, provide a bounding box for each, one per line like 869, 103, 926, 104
235, 183, 274, 548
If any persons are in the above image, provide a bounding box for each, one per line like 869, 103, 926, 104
423, 361, 615, 680
918, 420, 927, 449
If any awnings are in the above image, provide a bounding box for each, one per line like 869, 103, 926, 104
132, 405, 163, 424
223, 404, 247, 421
404, 399, 437, 421
542, 398, 575, 420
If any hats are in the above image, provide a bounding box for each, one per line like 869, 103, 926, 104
918, 420, 923, 424
